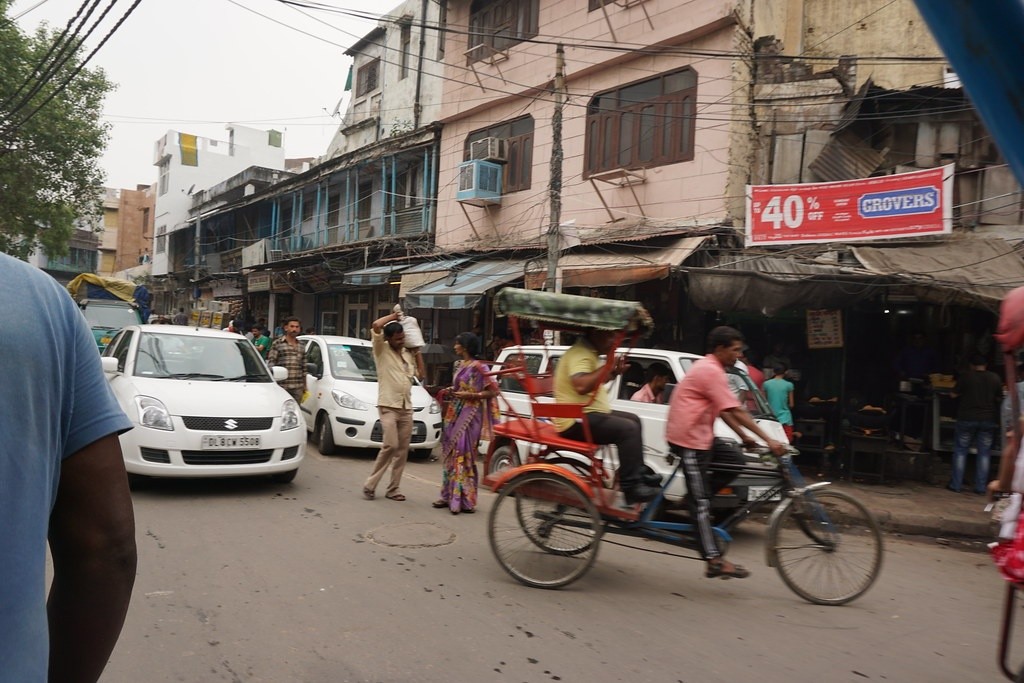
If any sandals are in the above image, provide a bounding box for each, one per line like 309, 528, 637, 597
393, 494, 405, 501
364, 487, 374, 499
706, 559, 750, 578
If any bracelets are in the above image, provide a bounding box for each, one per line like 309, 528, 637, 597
415, 350, 421, 356
479, 392, 482, 399
470, 393, 475, 400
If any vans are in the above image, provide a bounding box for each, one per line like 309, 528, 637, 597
476, 345, 792, 522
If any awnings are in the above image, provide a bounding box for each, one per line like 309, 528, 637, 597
524, 234, 710, 290
342, 255, 527, 309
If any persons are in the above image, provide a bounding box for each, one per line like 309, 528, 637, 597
667, 325, 802, 578
174, 307, 188, 325
159, 318, 173, 324
629, 362, 669, 403
307, 327, 316, 334
229, 321, 244, 334
0, 253, 139, 683
948, 352, 1024, 501
251, 323, 269, 361
432, 332, 500, 513
275, 319, 286, 338
253, 317, 265, 328
363, 313, 426, 501
267, 316, 307, 406
552, 326, 664, 506
151, 320, 161, 324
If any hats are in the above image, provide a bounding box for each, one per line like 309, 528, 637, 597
969, 355, 986, 366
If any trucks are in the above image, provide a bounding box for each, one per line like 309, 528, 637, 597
69, 273, 151, 358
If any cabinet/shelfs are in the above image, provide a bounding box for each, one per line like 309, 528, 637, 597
919, 383, 1006, 458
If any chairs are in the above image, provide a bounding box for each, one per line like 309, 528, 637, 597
622, 361, 643, 400
524, 373, 620, 508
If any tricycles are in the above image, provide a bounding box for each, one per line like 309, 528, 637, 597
475, 285, 884, 606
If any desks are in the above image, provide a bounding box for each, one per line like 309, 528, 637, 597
840, 430, 890, 485
885, 390, 932, 451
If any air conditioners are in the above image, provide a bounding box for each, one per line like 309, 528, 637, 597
458, 160, 501, 207
470, 137, 509, 164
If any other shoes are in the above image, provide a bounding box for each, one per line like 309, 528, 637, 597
623, 485, 664, 506
640, 472, 663, 486
433, 500, 448, 508
461, 506, 475, 513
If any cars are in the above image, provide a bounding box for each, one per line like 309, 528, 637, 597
99, 324, 307, 483
295, 335, 442, 459
